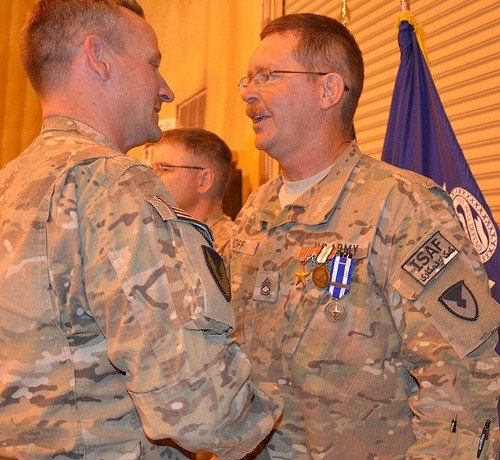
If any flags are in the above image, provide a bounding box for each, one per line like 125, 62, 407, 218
380, 17, 500, 355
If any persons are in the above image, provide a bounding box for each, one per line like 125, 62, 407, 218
149, 128, 242, 285
0, 0, 284, 460
232, 13, 500, 460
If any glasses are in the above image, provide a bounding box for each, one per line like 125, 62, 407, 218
237, 68, 350, 92
153, 163, 204, 178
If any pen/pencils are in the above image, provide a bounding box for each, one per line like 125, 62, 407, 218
477, 420, 491, 458
449, 414, 457, 433
